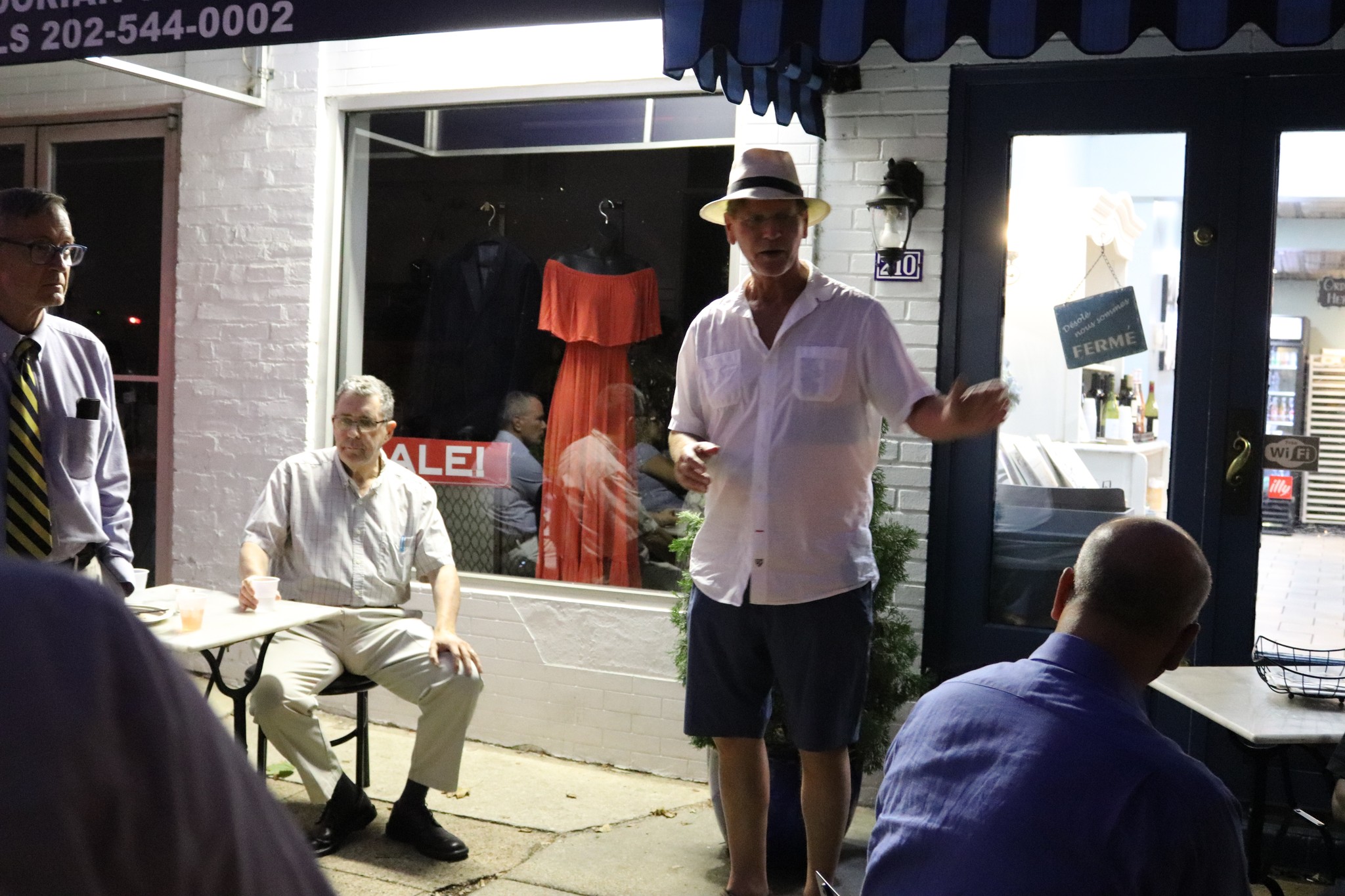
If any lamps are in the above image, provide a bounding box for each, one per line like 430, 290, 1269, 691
867, 155, 925, 278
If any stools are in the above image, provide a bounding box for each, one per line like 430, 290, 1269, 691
244, 657, 379, 788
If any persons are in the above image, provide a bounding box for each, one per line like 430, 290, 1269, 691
0, 188, 135, 595
238, 375, 485, 861
538, 234, 661, 589
669, 152, 1020, 895
861, 515, 1251, 896
0, 552, 337, 896
467, 383, 708, 593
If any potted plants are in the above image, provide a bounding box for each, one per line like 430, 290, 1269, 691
670, 412, 930, 888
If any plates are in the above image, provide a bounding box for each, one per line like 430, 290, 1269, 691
126, 602, 174, 626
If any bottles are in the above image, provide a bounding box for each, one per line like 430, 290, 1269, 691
1143, 380, 1160, 440
1266, 397, 1296, 422
1087, 373, 1145, 438
1262, 467, 1290, 491
1268, 345, 1298, 369
1269, 372, 1280, 391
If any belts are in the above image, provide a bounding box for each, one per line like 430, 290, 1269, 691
502, 532, 535, 554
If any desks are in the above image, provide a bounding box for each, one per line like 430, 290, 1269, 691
1071, 438, 1170, 519
1148, 665, 1345, 896
121, 582, 344, 762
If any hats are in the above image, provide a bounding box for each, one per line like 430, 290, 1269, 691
698, 148, 832, 227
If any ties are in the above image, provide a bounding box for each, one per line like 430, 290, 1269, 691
5, 338, 53, 562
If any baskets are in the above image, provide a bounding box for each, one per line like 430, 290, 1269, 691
1251, 636, 1345, 704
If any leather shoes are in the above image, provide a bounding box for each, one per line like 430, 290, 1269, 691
307, 787, 377, 859
385, 800, 469, 860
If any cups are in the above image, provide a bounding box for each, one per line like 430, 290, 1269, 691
127, 568, 150, 602
181, 607, 202, 632
246, 576, 281, 615
1082, 397, 1135, 441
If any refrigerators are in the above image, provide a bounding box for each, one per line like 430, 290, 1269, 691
1260, 314, 1310, 538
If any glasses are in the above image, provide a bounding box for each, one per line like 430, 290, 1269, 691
332, 414, 389, 433
0, 235, 88, 267
649, 416, 667, 426
